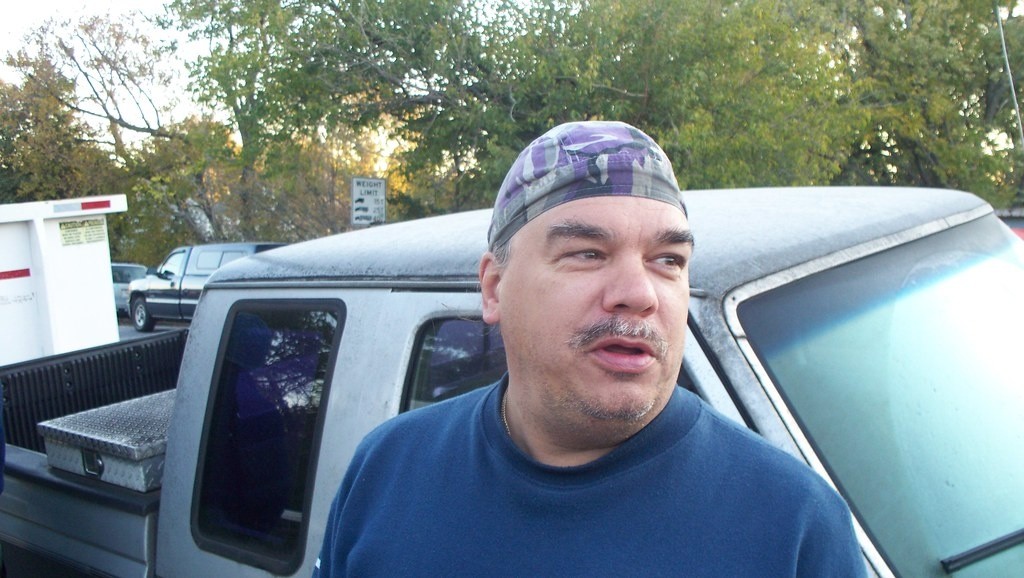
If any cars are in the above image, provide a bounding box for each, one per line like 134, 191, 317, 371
112, 263, 149, 311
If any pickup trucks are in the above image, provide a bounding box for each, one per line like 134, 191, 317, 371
0, 183, 1023, 578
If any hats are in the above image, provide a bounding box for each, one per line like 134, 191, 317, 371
486, 120, 688, 256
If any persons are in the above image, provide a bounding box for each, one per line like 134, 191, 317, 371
307, 120, 870, 578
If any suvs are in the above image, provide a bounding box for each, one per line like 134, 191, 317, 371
128, 242, 289, 332
